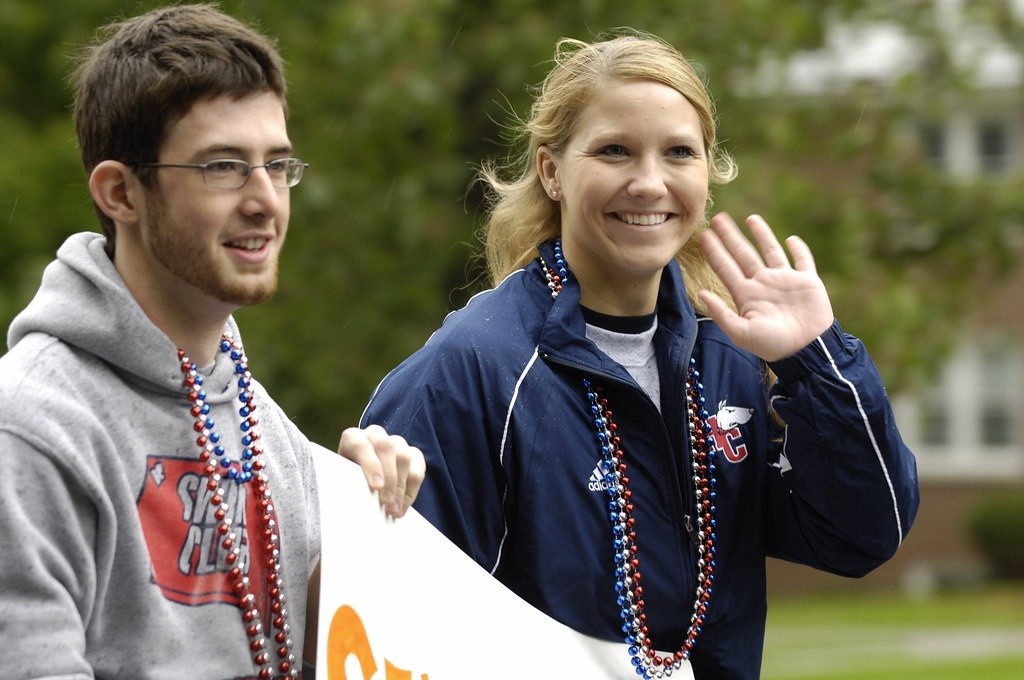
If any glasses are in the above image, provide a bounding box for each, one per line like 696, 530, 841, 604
150, 159, 309, 190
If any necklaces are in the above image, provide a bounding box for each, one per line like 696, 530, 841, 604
174, 333, 304, 680
538, 234, 720, 680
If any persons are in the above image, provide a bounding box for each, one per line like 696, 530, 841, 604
359, 36, 920, 680
0, 3, 428, 680
